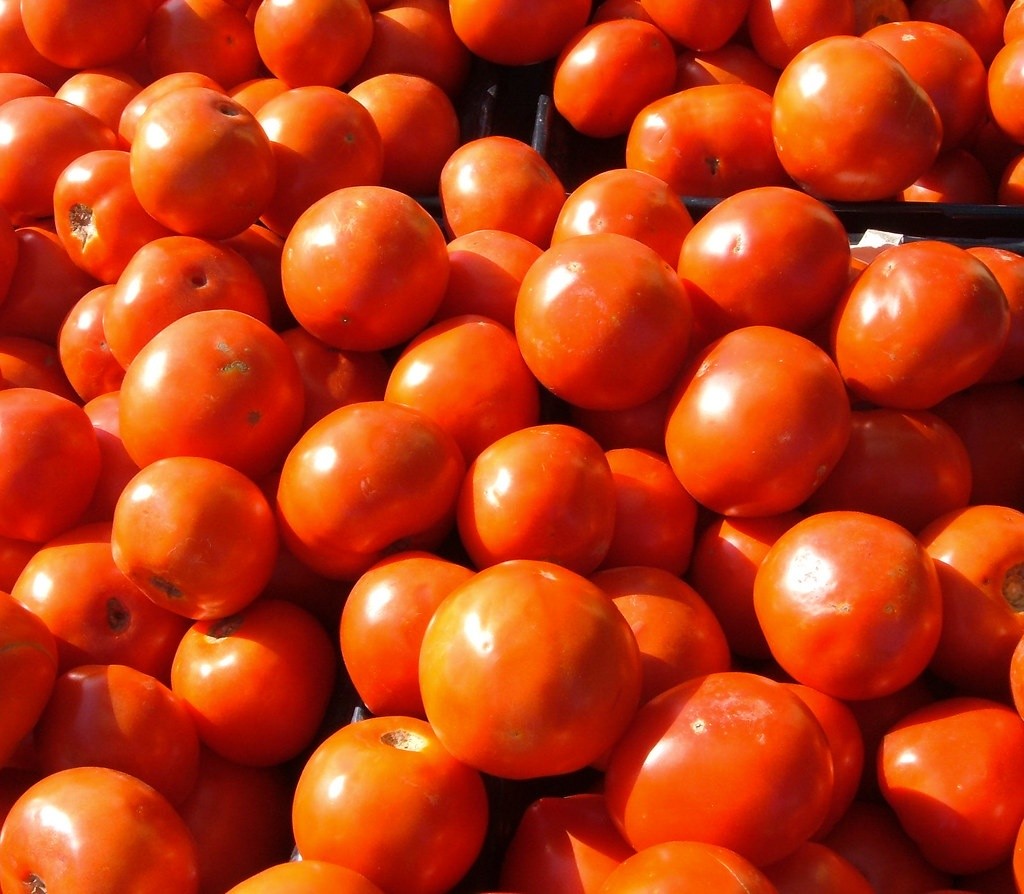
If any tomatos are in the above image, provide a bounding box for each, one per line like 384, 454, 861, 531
0, 0, 1024, 894
